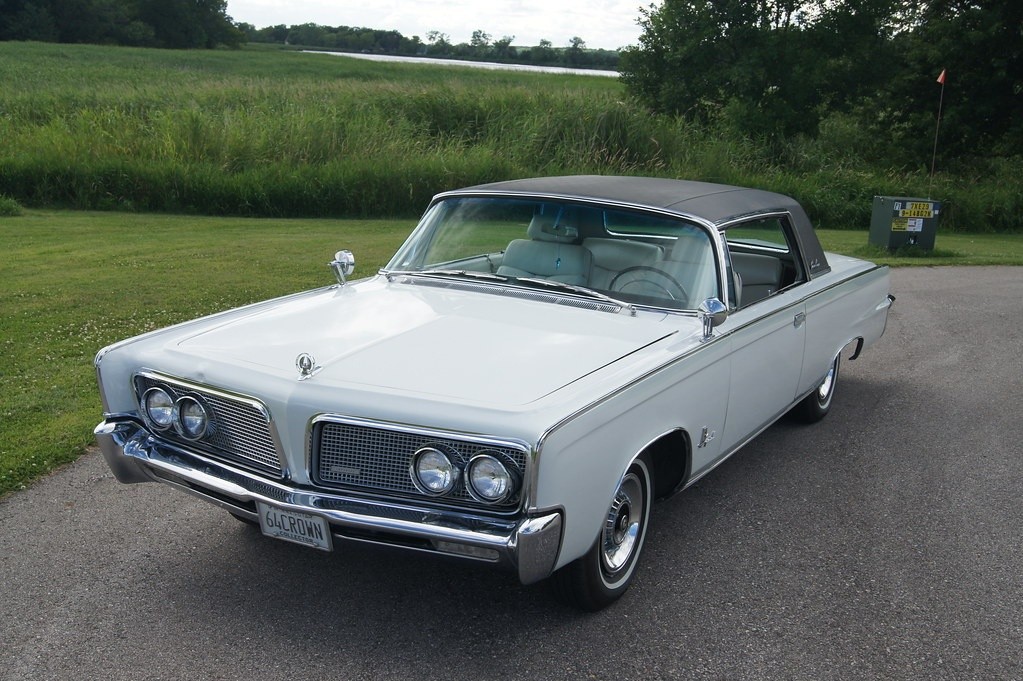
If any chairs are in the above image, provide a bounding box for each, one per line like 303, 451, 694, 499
581, 237, 663, 297
640, 236, 742, 311
730, 252, 782, 307
495, 203, 592, 289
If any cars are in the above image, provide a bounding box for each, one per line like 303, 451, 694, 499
98, 177, 896, 596
